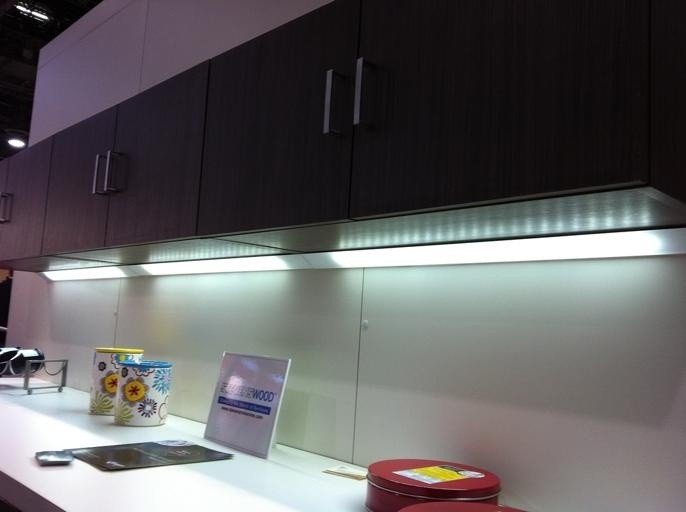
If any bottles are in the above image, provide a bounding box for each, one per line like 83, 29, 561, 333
0, 345, 46, 377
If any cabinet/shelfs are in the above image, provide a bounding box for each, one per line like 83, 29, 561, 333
41, 60, 299, 267
516, 0, 686, 237
0, 136, 56, 272
197, 0, 517, 237
